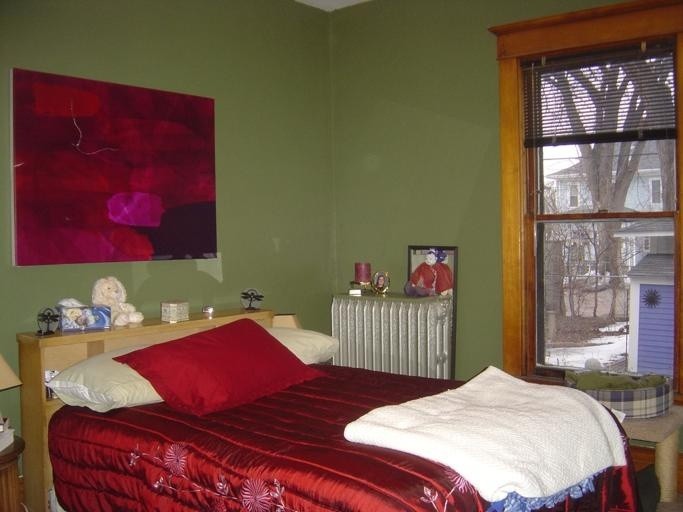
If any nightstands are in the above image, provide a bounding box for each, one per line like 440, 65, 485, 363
1, 435, 26, 512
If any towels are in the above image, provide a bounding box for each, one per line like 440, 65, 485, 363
342, 365, 628, 502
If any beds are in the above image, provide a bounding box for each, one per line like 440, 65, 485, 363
19, 305, 639, 512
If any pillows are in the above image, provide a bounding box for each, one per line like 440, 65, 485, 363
111, 318, 329, 416
45, 342, 164, 412
266, 326, 340, 367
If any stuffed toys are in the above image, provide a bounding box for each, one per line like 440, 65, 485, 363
91, 276, 144, 327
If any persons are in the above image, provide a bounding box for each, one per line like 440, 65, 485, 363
405, 249, 451, 296
375, 275, 384, 291
435, 249, 453, 287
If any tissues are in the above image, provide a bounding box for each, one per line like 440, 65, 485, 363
54, 298, 112, 332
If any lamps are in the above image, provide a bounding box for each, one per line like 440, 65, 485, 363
0, 353, 24, 394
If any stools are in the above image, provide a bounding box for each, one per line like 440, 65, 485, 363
620, 406, 683, 503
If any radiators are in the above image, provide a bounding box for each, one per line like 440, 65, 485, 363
329, 293, 452, 381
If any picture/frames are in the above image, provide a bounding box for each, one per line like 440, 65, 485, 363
408, 244, 457, 379
